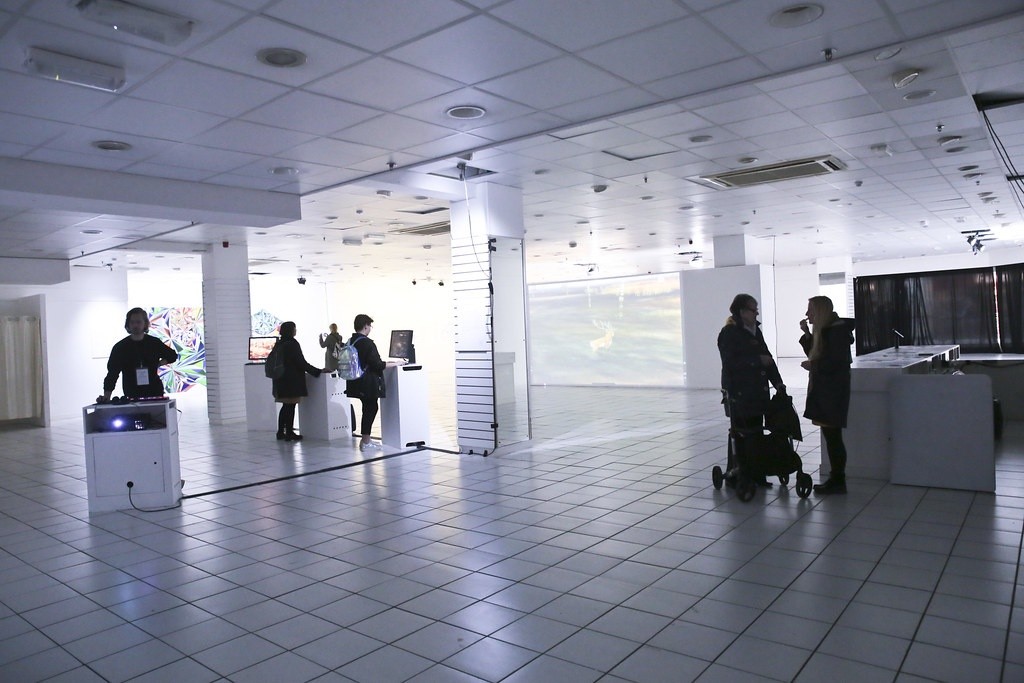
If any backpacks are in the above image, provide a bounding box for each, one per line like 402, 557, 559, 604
332, 335, 342, 359
264, 341, 292, 378
337, 337, 370, 380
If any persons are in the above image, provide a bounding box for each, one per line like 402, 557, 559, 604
272, 321, 332, 442
346, 314, 408, 452
798, 296, 857, 494
319, 323, 342, 370
96, 308, 178, 404
718, 293, 786, 487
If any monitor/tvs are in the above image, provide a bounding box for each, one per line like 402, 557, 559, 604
248, 336, 279, 359
389, 330, 416, 365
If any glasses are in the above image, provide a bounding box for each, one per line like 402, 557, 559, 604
745, 306, 759, 313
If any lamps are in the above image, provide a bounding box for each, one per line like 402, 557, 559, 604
973, 241, 985, 255
891, 69, 918, 90
76, 0, 199, 47
967, 235, 976, 246
15, 47, 125, 94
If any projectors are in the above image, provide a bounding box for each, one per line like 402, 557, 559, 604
109, 413, 151, 430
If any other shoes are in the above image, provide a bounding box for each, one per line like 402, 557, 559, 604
285, 431, 303, 441
277, 430, 285, 440
360, 439, 383, 451
813, 470, 847, 494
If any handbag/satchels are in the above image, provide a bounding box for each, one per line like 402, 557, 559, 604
765, 388, 803, 442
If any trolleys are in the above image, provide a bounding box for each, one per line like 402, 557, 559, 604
713, 389, 813, 503
